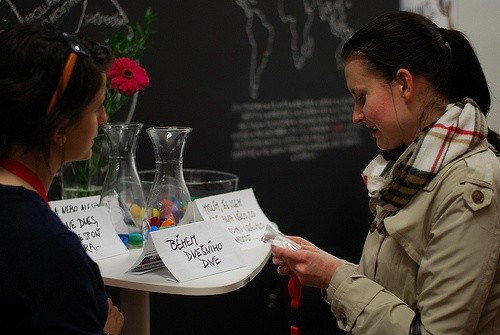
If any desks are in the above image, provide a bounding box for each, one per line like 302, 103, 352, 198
102, 221, 279, 335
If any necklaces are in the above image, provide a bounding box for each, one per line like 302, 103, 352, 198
3, 158, 50, 206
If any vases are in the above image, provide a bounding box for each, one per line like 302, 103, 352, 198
62, 126, 108, 200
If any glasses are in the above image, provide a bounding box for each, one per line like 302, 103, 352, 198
44, 22, 91, 114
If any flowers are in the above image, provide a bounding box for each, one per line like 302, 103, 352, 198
68, 9, 152, 190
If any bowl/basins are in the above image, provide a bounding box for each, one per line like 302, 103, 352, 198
139, 169, 238, 201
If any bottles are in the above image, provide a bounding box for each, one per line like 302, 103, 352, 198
99, 123, 146, 268
142, 126, 194, 249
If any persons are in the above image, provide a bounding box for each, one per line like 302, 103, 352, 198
0, 21, 126, 335
271, 10, 500, 335
